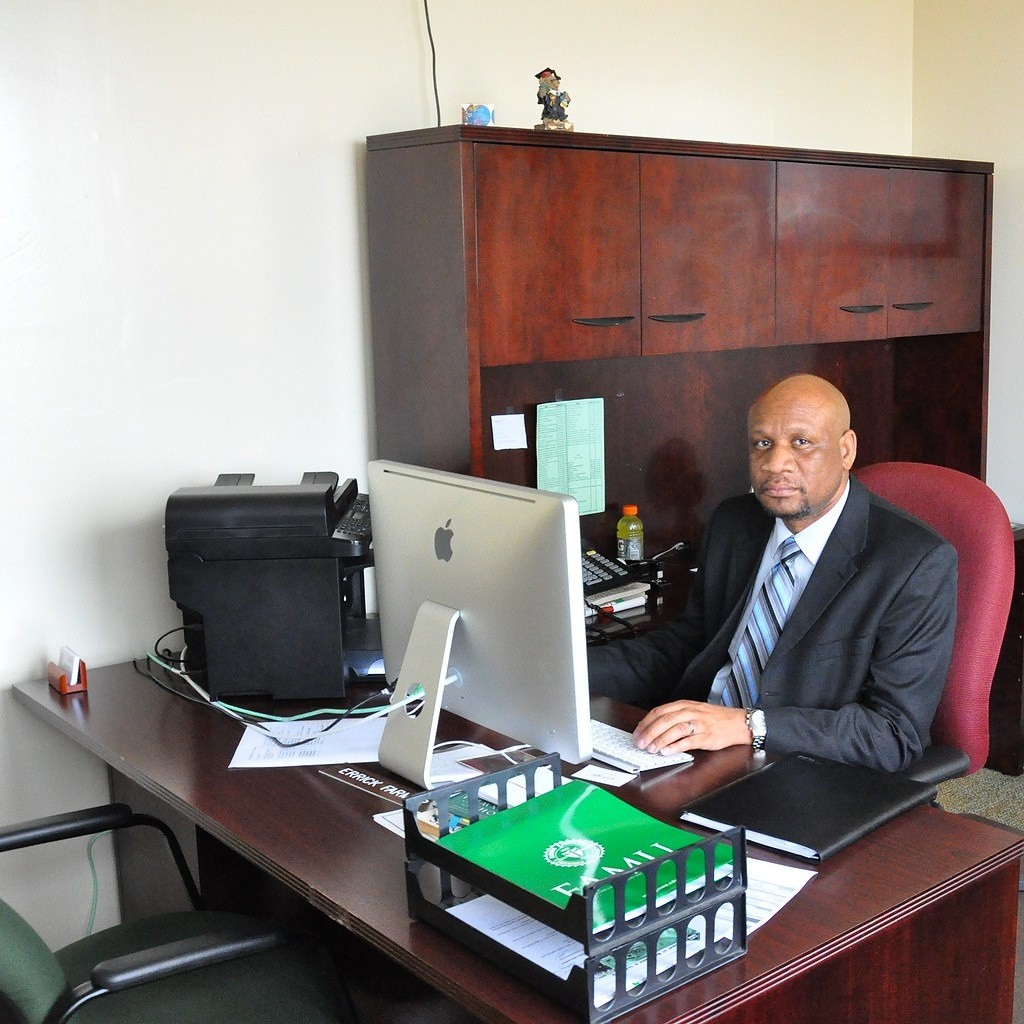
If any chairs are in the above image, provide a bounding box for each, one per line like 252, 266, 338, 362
853, 461, 1016, 814
0, 803, 358, 1024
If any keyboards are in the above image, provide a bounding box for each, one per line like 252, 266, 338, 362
590, 719, 694, 774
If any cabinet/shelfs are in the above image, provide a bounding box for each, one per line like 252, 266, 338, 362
401, 752, 747, 1024
366, 124, 1023, 646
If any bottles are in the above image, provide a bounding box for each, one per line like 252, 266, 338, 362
616, 504, 644, 561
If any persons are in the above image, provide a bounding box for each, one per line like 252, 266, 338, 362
585, 372, 958, 775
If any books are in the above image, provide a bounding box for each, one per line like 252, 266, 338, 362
434, 780, 736, 936
678, 752, 937, 862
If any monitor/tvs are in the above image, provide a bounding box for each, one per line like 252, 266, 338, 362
365, 457, 593, 792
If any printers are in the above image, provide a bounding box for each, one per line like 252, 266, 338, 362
164, 471, 387, 701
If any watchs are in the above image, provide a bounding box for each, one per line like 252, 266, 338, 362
745, 706, 766, 751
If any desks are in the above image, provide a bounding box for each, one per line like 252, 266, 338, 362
9, 652, 1024, 1023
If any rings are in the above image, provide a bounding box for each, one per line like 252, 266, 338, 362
686, 721, 695, 736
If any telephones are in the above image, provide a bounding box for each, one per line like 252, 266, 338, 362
581, 536, 634, 596
